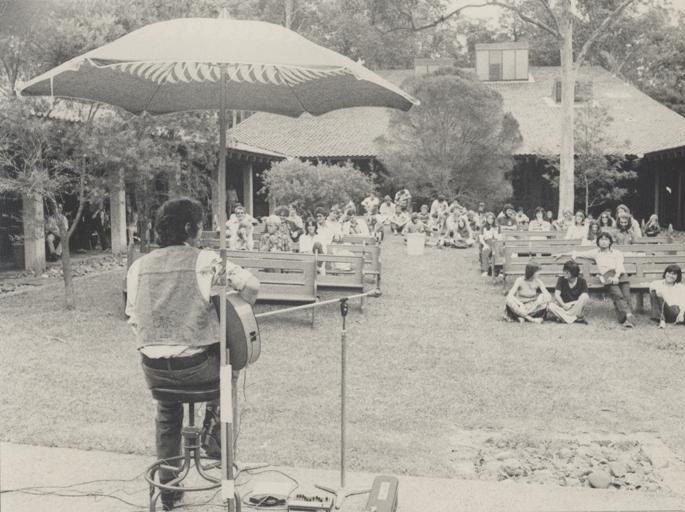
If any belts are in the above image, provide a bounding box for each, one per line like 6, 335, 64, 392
142, 351, 210, 371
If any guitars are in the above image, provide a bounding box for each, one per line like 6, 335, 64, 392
213, 294, 261, 372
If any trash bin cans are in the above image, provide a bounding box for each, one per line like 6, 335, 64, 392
405, 232, 426, 258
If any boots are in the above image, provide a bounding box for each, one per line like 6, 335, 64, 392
159, 480, 185, 510
200, 403, 222, 458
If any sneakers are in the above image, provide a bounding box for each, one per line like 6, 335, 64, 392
624, 321, 634, 327
534, 318, 544, 324
658, 320, 666, 328
517, 317, 526, 322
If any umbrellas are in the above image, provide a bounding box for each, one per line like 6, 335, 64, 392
20, 8, 420, 512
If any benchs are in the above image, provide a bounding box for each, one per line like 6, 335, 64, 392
491, 231, 685, 313
200, 238, 380, 329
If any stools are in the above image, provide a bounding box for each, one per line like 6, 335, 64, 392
144, 390, 241, 512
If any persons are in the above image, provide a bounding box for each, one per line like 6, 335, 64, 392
210, 185, 388, 275
504, 203, 685, 329
46, 202, 70, 261
380, 186, 519, 278
123, 198, 261, 511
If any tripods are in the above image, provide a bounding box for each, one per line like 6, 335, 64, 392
257, 288, 381, 511
